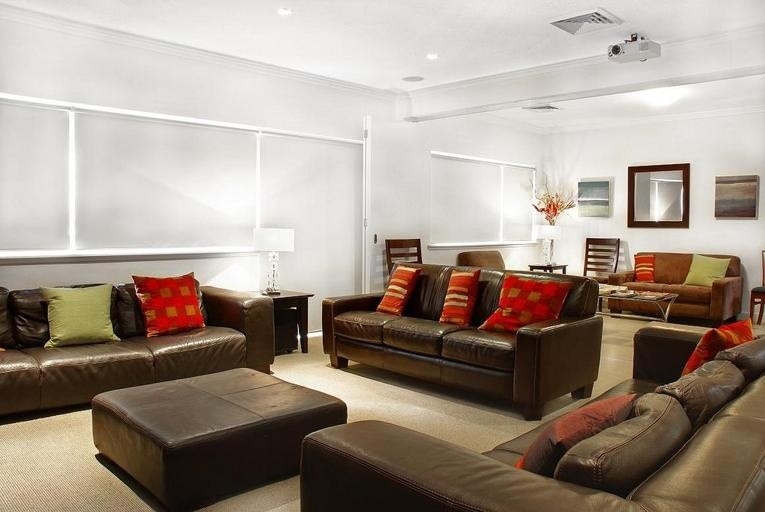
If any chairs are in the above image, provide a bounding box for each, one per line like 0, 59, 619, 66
750, 249, 765, 325
583, 237, 621, 311
385, 238, 423, 276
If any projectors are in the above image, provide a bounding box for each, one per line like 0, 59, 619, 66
607, 31, 662, 65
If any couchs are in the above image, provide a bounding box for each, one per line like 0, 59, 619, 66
607, 251, 744, 328
0, 278, 275, 416
90, 366, 348, 510
321, 261, 604, 421
299, 326, 765, 512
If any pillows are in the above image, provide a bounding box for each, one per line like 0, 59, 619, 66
634, 254, 656, 283
130, 270, 207, 339
680, 317, 753, 378
439, 268, 482, 327
375, 264, 424, 317
477, 273, 577, 334
683, 254, 731, 288
39, 283, 122, 350
514, 391, 638, 479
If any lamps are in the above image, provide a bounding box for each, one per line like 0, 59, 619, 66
252, 226, 295, 295
536, 225, 561, 266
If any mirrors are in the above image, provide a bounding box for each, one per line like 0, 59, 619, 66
627, 162, 690, 229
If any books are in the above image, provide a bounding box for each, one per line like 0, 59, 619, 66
607, 289, 670, 302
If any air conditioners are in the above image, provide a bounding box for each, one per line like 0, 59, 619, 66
548, 7, 624, 37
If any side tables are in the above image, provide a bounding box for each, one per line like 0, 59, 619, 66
249, 289, 315, 354
528, 263, 568, 274
